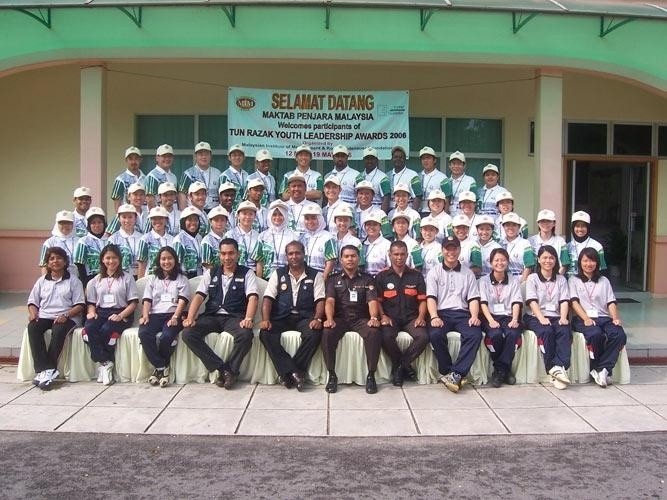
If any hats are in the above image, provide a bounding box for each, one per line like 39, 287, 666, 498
194, 141, 212, 153
156, 143, 174, 155
55, 173, 410, 226
390, 145, 406, 153
361, 147, 378, 160
124, 146, 141, 159
295, 144, 312, 154
442, 236, 460, 248
420, 151, 591, 230
418, 146, 437, 157
228, 144, 245, 155
255, 149, 273, 162
332, 145, 349, 156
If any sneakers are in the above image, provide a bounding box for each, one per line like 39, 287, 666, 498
39, 369, 60, 391
590, 367, 609, 388
148, 368, 159, 386
96, 364, 103, 383
157, 365, 170, 388
546, 375, 567, 390
103, 361, 114, 385
441, 371, 461, 392
32, 372, 39, 386
607, 376, 615, 385
549, 365, 571, 384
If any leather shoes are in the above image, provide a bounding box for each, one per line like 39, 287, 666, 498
366, 374, 376, 394
492, 373, 505, 388
291, 373, 306, 392
505, 372, 516, 385
221, 368, 234, 389
216, 372, 240, 386
283, 376, 294, 389
325, 374, 338, 393
405, 365, 417, 379
391, 364, 404, 386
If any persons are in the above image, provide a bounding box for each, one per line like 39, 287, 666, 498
523, 245, 573, 390
409, 145, 607, 282
28, 246, 87, 388
39, 141, 278, 278
425, 235, 482, 393
250, 144, 424, 278
475, 248, 523, 388
320, 245, 383, 394
137, 245, 191, 387
374, 241, 430, 386
568, 247, 627, 388
85, 244, 139, 385
181, 238, 259, 388
260, 240, 326, 391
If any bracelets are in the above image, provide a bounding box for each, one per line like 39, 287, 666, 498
430, 317, 440, 322
117, 314, 123, 320
380, 313, 386, 316
245, 317, 253, 323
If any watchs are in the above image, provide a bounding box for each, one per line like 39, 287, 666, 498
61, 312, 70, 319
370, 316, 379, 321
313, 318, 324, 323
172, 315, 180, 320
